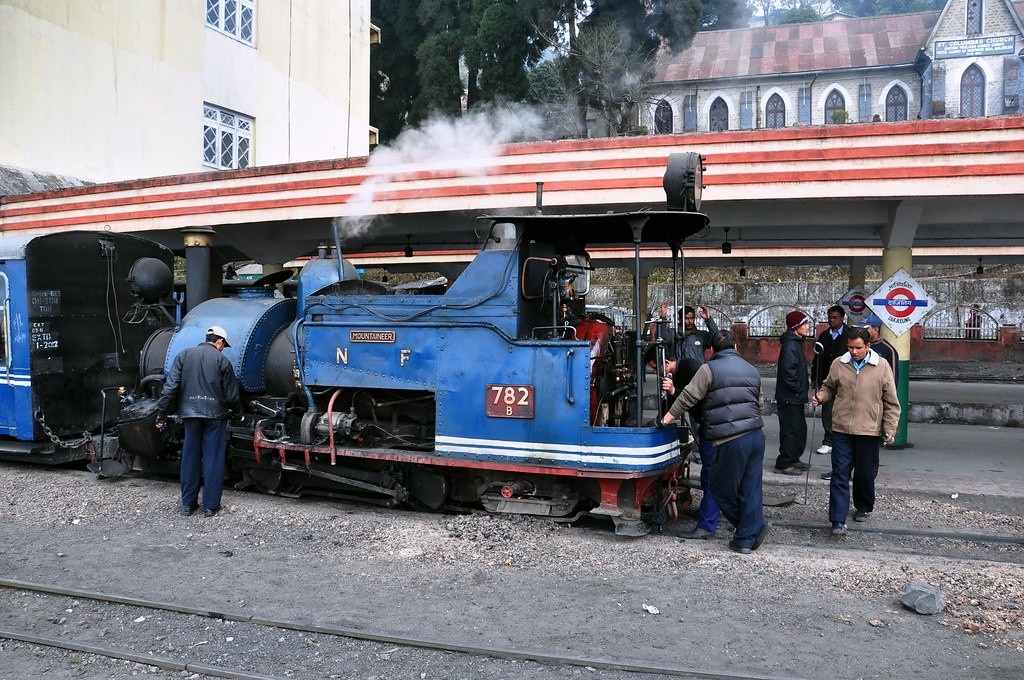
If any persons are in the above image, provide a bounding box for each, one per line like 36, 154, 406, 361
960, 304, 981, 338
810, 305, 851, 454
812, 327, 902, 537
644, 300, 770, 554
155, 326, 243, 516
821, 314, 899, 479
775, 311, 812, 475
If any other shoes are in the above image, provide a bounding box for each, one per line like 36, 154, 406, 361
751, 520, 768, 550
677, 524, 717, 540
204, 508, 220, 516
794, 460, 811, 471
821, 470, 834, 480
729, 537, 750, 554
817, 444, 832, 454
773, 466, 801, 476
693, 449, 703, 465
854, 509, 868, 522
831, 520, 846, 536
182, 504, 199, 515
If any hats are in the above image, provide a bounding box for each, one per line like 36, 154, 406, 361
850, 314, 882, 330
785, 311, 809, 330
206, 325, 232, 348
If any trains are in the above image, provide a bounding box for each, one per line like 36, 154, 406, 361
0, 153, 711, 539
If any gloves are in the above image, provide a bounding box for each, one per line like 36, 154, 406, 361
234, 411, 247, 427
648, 418, 664, 429
